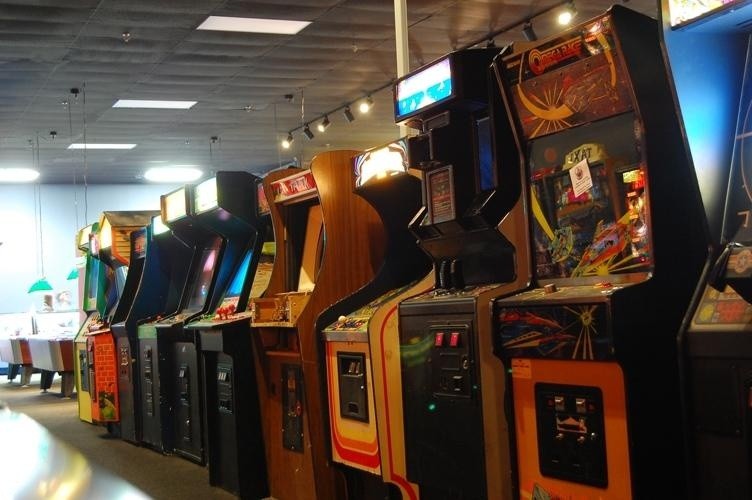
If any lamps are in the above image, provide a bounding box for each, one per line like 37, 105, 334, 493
29, 130, 52, 298
278, 0, 576, 150
63, 98, 80, 281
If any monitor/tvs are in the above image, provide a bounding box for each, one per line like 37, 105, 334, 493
88, 254, 100, 300
184, 246, 218, 305
112, 266, 126, 298
225, 249, 252, 296
524, 157, 654, 284
